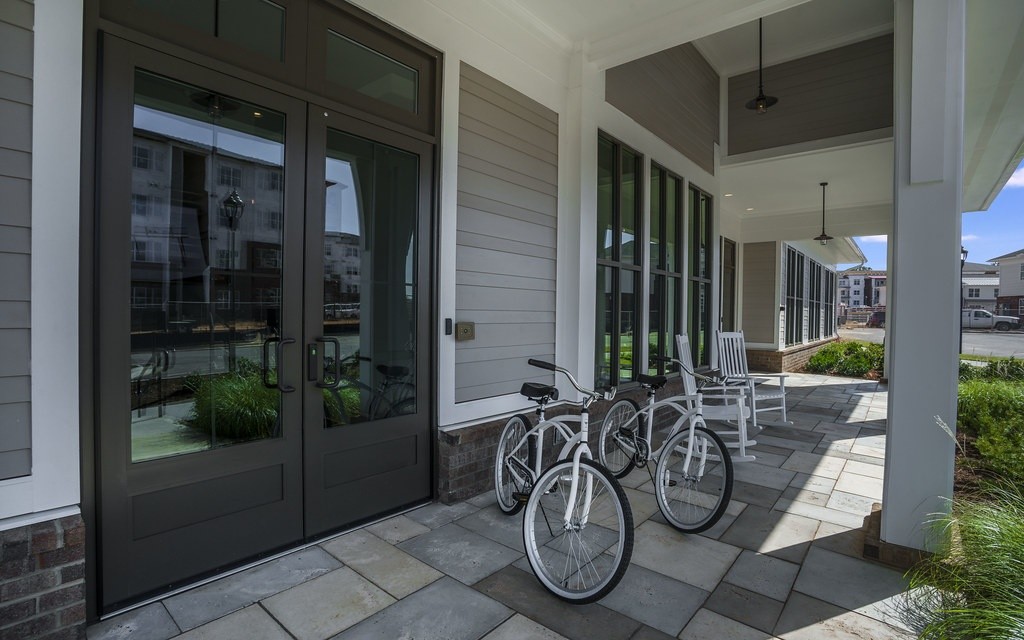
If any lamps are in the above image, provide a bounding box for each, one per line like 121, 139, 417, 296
814, 183, 834, 246
745, 18, 779, 115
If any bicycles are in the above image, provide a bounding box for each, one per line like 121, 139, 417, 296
494, 358, 635, 605
598, 355, 734, 535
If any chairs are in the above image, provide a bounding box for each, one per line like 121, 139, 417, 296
714, 329, 794, 431
662, 333, 757, 463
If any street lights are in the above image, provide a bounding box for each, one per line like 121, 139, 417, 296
223, 188, 246, 373
959, 244, 969, 355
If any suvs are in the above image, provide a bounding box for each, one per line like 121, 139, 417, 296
868, 311, 885, 328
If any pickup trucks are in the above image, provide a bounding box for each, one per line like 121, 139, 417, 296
961, 309, 1022, 331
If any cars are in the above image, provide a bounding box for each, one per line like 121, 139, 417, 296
324, 302, 361, 320
